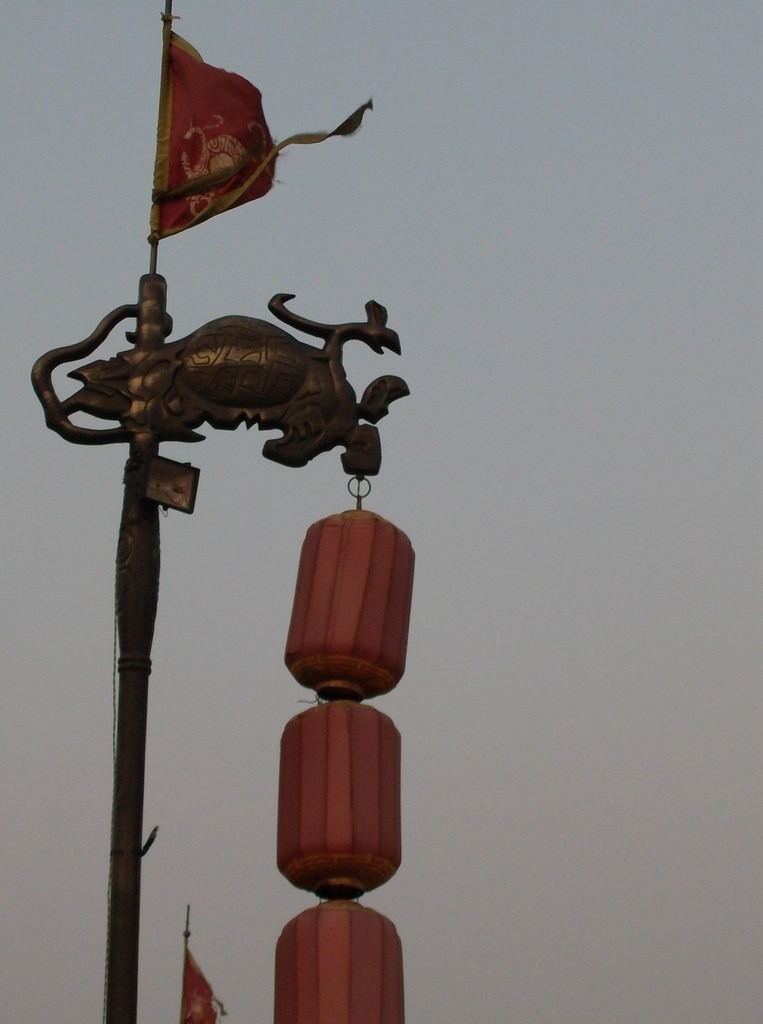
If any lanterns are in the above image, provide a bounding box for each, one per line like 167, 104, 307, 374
277, 702, 402, 898
272, 903, 407, 1024
285, 506, 416, 698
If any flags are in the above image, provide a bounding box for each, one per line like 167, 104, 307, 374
180, 949, 227, 1024
149, 26, 373, 241
